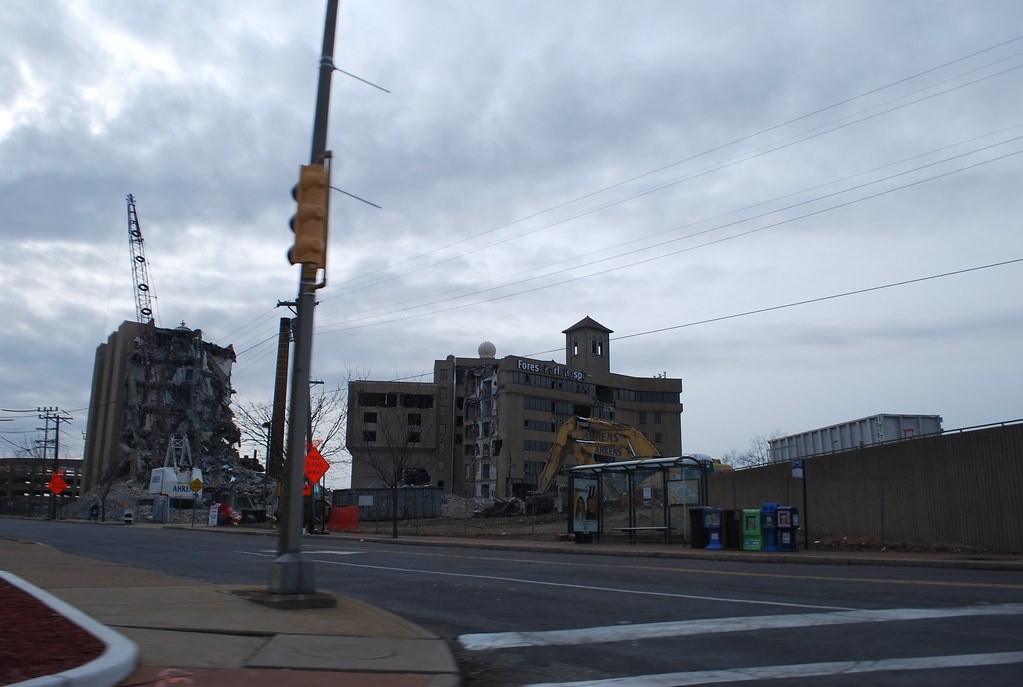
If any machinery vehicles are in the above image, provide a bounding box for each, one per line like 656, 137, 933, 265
525, 416, 735, 535
123, 192, 205, 507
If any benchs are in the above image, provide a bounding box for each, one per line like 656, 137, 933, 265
610, 526, 676, 544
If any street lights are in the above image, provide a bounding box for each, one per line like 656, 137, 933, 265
792, 458, 808, 549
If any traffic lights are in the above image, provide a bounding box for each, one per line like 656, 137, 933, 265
285, 163, 328, 267
